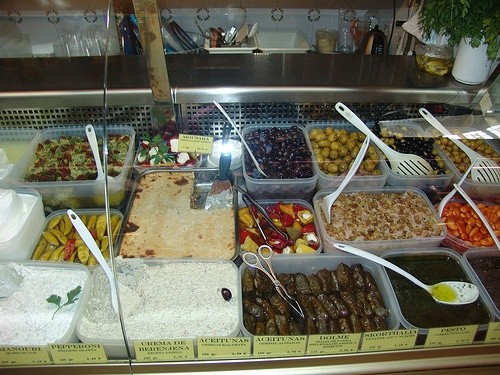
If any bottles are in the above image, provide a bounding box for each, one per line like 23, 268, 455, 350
121, 16, 138, 56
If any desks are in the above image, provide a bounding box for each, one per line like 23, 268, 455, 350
0, 53, 500, 140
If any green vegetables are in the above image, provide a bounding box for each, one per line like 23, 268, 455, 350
46, 286, 81, 320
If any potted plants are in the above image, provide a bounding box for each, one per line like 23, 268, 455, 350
418, 0, 500, 85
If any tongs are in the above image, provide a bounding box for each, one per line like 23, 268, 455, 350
242, 194, 289, 248
243, 245, 306, 323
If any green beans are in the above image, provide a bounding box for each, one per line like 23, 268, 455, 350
31, 215, 121, 265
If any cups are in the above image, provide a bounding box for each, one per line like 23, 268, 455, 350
316, 29, 339, 54
339, 8, 355, 52
